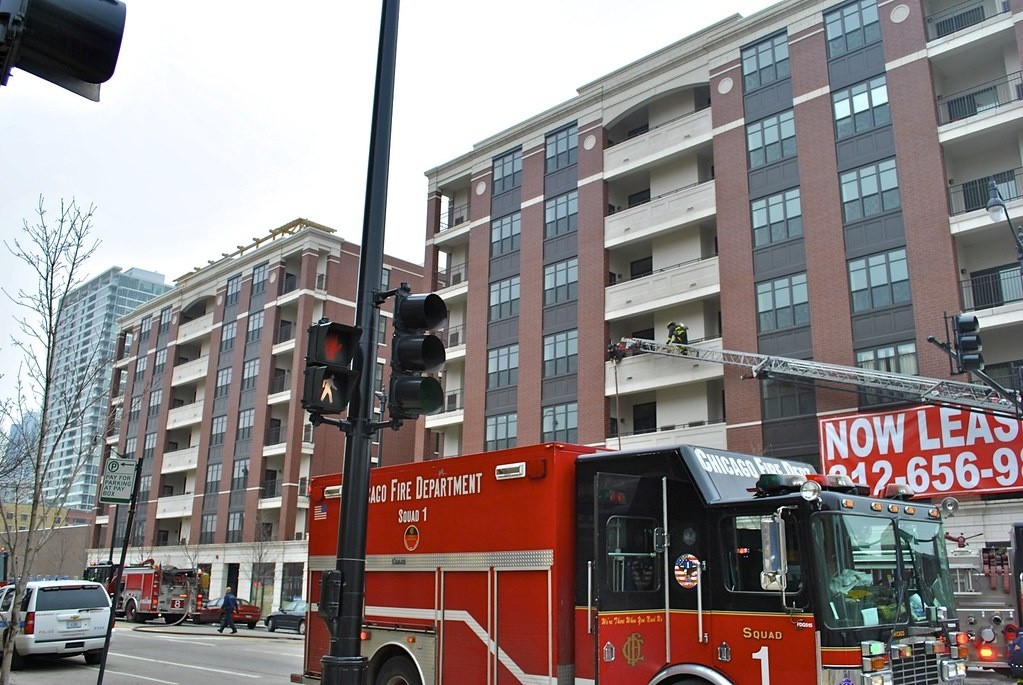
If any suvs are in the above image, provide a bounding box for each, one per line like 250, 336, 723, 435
0, 580, 114, 665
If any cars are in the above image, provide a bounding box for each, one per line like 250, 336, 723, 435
202, 597, 260, 630
264, 601, 305, 634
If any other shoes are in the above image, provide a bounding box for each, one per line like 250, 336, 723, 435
230, 630, 237, 634
218, 629, 222, 633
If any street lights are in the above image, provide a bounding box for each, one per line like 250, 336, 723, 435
985, 175, 1022, 280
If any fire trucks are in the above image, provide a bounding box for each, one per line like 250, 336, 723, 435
610, 334, 1023, 676
85, 561, 203, 623
291, 443, 968, 684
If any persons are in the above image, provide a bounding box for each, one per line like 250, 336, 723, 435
218, 587, 239, 634
1008, 630, 1023, 685
665, 322, 688, 355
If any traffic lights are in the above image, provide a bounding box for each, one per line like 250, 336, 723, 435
0, 0, 127, 103
304, 318, 363, 415
389, 281, 447, 420
952, 315, 985, 372
